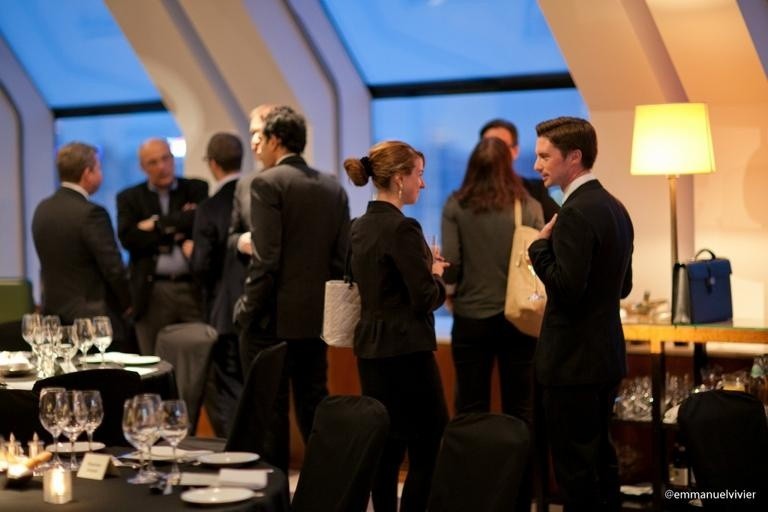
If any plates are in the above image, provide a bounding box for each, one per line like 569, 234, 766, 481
197, 451, 260, 464
79, 352, 114, 364
115, 355, 161, 364
47, 441, 106, 454
181, 487, 254, 504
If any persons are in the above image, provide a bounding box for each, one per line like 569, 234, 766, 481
182, 131, 244, 437
480, 118, 561, 225
526, 117, 635, 512
229, 104, 349, 470
31, 141, 132, 354
116, 138, 208, 355
343, 138, 450, 512
439, 137, 544, 418
209, 101, 283, 448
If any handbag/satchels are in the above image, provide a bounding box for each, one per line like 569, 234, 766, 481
320, 217, 363, 349
504, 194, 547, 338
671, 247, 734, 326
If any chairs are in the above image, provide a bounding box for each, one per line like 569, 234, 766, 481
423, 411, 536, 511
290, 394, 392, 511
653, 391, 768, 512
221, 340, 289, 451
154, 322, 218, 436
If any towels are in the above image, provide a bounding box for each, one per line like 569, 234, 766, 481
216, 467, 268, 490
144, 445, 215, 464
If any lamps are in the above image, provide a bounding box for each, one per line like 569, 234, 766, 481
631, 103, 715, 346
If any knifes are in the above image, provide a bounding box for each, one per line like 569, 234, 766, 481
163, 478, 173, 495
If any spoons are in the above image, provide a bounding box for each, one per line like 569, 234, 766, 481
148, 479, 166, 494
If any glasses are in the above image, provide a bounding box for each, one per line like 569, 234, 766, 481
204, 156, 211, 161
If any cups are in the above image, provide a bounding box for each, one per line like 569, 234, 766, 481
73, 318, 93, 371
32, 326, 53, 377
21, 314, 39, 356
74, 390, 102, 453
424, 235, 436, 261
158, 400, 189, 487
37, 387, 69, 469
57, 326, 79, 374
55, 392, 87, 472
92, 316, 113, 369
133, 393, 163, 478
43, 316, 61, 347
122, 401, 157, 483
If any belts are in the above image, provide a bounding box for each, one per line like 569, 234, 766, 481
154, 272, 189, 282
